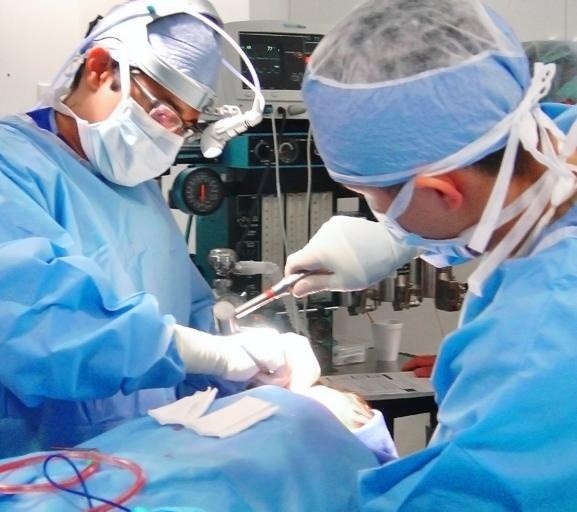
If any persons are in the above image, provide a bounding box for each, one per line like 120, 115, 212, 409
0, 0, 291, 459
311, 384, 375, 432
284, 0, 577, 512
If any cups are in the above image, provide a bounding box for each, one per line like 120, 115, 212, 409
370, 319, 404, 361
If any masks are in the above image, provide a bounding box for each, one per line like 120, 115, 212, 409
371, 190, 478, 269
75, 90, 183, 187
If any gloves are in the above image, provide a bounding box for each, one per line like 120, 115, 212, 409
284, 215, 426, 298
173, 325, 291, 389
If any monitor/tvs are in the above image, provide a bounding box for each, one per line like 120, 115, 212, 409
223, 18, 325, 124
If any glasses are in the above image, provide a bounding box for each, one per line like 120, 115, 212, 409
130, 73, 195, 141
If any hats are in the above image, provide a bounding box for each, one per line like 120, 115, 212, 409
354, 409, 399, 461
94, 2, 220, 86
